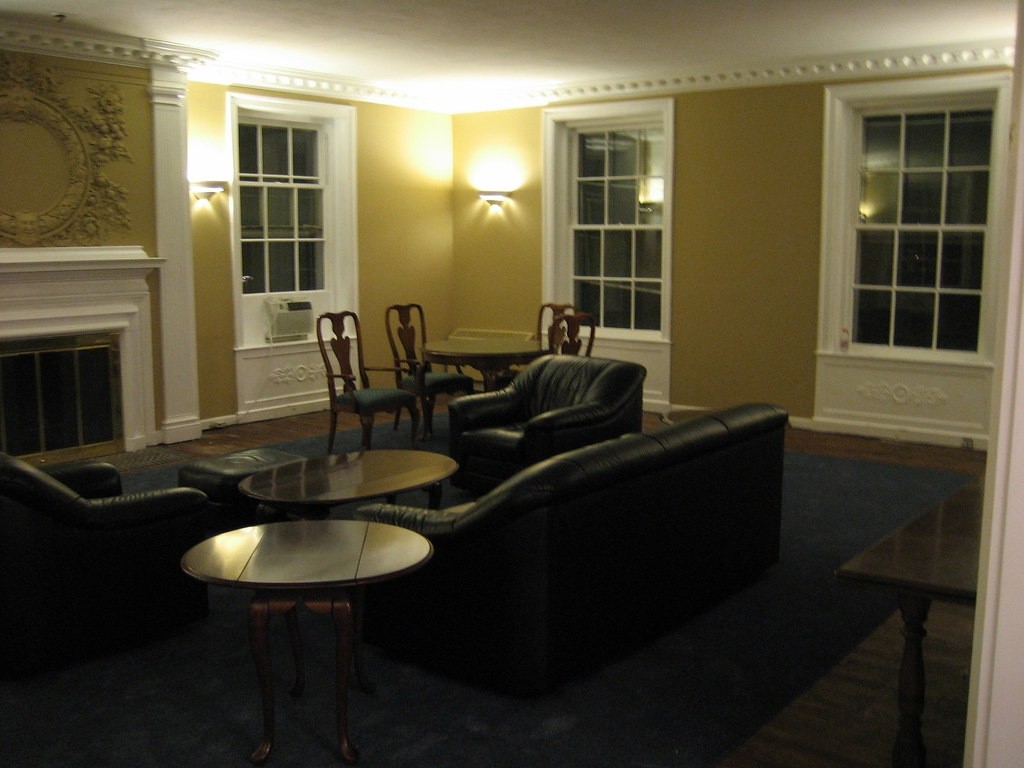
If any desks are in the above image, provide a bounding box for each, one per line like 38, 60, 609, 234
419, 342, 558, 393
834, 473, 984, 768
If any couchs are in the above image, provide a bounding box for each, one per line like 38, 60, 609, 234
352, 404, 788, 708
447, 355, 647, 497
0, 450, 209, 684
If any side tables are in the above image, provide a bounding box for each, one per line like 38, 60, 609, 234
180, 520, 435, 768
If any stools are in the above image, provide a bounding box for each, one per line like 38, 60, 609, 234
179, 446, 308, 538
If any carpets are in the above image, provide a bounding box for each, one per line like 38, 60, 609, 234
0, 412, 974, 768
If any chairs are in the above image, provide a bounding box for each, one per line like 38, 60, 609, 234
385, 302, 474, 440
549, 312, 596, 356
316, 310, 421, 453
504, 304, 579, 388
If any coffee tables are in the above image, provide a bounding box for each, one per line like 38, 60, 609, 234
238, 449, 459, 525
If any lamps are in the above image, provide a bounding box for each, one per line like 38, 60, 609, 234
637, 202, 663, 214
476, 190, 513, 208
188, 180, 227, 201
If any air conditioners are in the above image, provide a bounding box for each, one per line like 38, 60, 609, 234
449, 328, 534, 382
